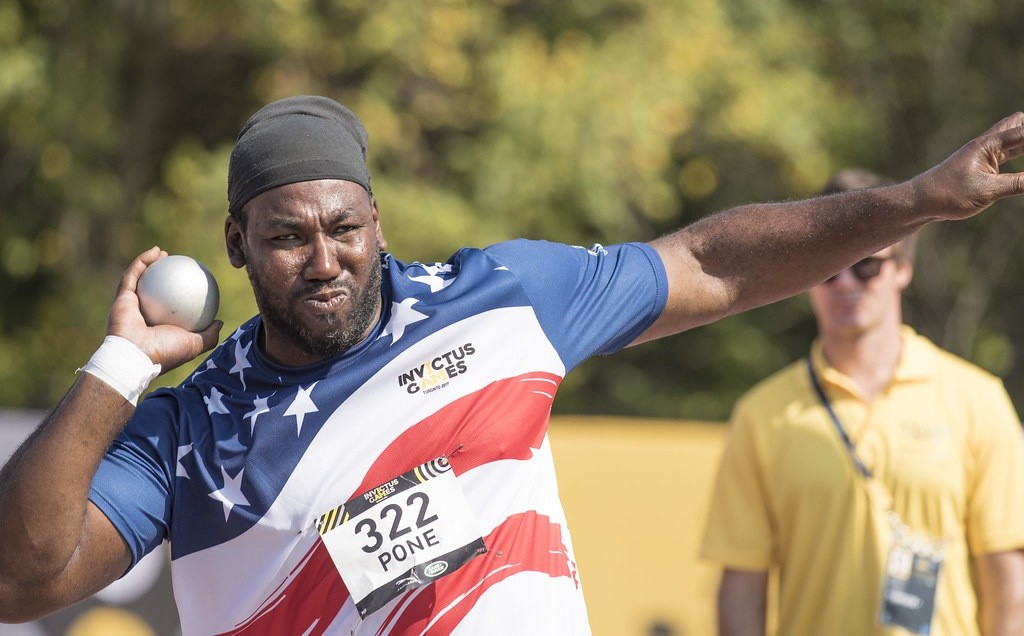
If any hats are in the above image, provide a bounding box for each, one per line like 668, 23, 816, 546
225, 95, 372, 214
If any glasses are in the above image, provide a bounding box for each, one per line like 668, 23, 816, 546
821, 255, 898, 282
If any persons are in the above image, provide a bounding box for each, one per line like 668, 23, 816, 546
0, 96, 1024, 636
695, 168, 1024, 635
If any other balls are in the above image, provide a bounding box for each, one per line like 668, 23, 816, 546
135, 253, 223, 333
